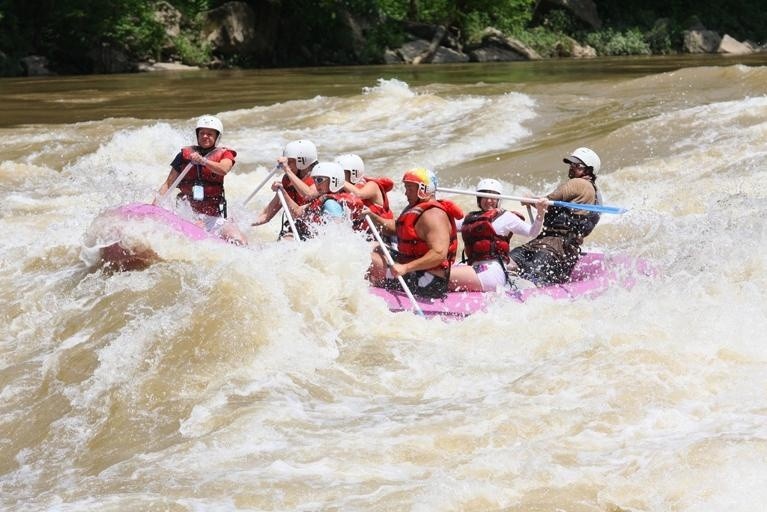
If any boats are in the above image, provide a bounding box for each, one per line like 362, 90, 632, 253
87, 203, 659, 322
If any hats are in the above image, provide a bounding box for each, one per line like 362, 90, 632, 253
562, 155, 580, 165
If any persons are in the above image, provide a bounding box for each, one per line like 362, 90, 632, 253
448, 179, 550, 292
336, 153, 394, 231
506, 146, 603, 287
360, 167, 465, 299
247, 139, 317, 240
272, 162, 369, 240
151, 116, 244, 247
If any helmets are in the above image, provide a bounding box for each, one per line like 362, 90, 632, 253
402, 167, 439, 201
572, 147, 601, 177
284, 139, 319, 171
196, 114, 224, 136
476, 177, 504, 195
311, 161, 347, 193
335, 153, 366, 183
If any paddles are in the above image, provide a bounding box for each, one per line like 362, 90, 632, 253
436, 187, 631, 216
